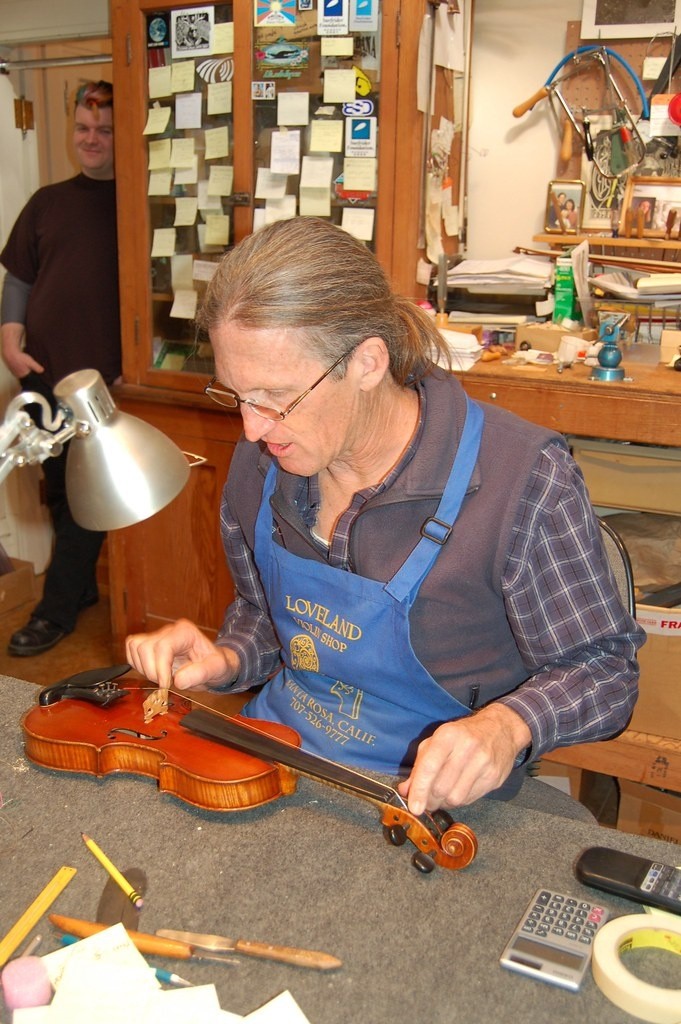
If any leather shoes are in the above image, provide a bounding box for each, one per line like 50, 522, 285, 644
6, 614, 65, 656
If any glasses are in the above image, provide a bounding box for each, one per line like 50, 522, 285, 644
204, 336, 362, 423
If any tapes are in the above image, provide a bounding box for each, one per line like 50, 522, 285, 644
591, 910, 681, 1024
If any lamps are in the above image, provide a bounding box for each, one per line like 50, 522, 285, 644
1, 371, 192, 532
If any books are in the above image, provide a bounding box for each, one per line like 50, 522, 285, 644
429, 253, 681, 345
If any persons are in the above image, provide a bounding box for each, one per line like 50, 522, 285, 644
550, 192, 577, 229
633, 199, 651, 229
1, 80, 122, 656
125, 217, 647, 816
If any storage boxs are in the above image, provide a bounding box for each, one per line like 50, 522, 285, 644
615, 776, 681, 846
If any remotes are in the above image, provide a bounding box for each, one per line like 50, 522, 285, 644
576, 846, 681, 918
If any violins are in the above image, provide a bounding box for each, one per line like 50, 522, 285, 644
20, 664, 479, 873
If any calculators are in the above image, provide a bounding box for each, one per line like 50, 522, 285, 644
498, 885, 610, 996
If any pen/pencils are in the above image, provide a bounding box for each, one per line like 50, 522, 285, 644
79, 831, 144, 909
50, 931, 193, 987
19, 933, 43, 961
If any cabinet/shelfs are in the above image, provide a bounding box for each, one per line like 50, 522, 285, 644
98, 0, 680, 796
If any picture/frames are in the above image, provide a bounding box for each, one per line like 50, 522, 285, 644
619, 176, 681, 241
541, 180, 587, 233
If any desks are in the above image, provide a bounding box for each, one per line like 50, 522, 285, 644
1, 673, 680, 1023
